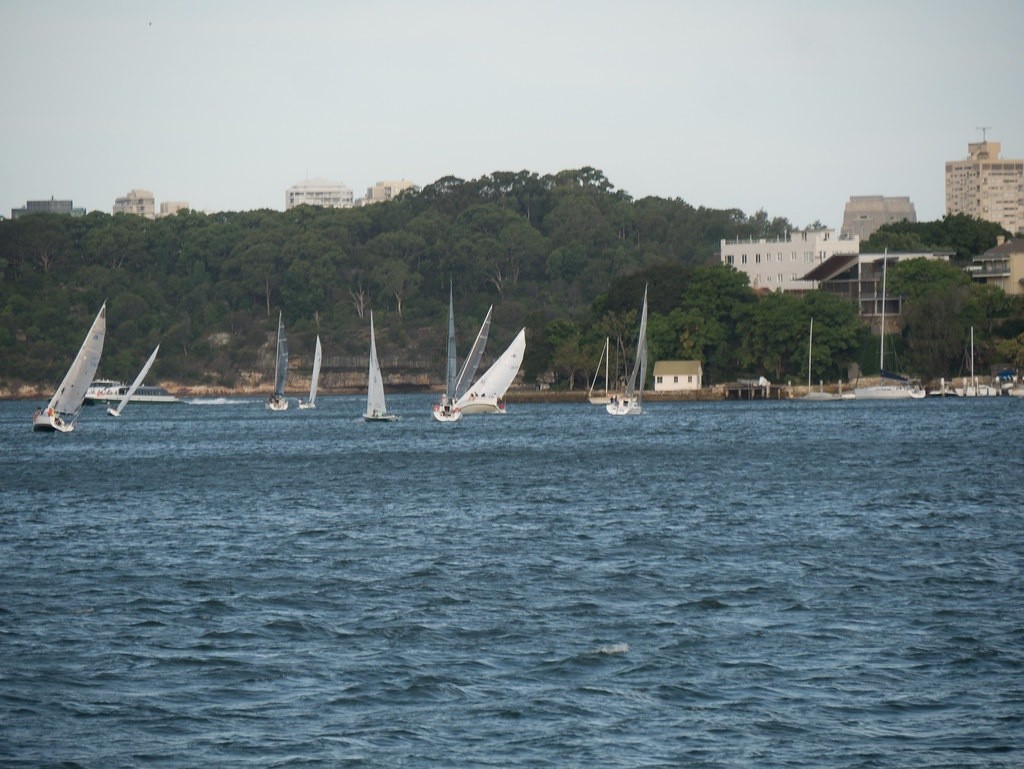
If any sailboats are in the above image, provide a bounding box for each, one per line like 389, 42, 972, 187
785, 318, 855, 400
928, 326, 997, 396
265, 309, 289, 410
298, 334, 322, 409
584, 283, 650, 416
362, 309, 398, 422
107, 344, 160, 417
836, 247, 927, 400
32, 299, 107, 433
432, 281, 526, 422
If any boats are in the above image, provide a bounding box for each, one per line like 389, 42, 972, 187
85, 379, 180, 403
